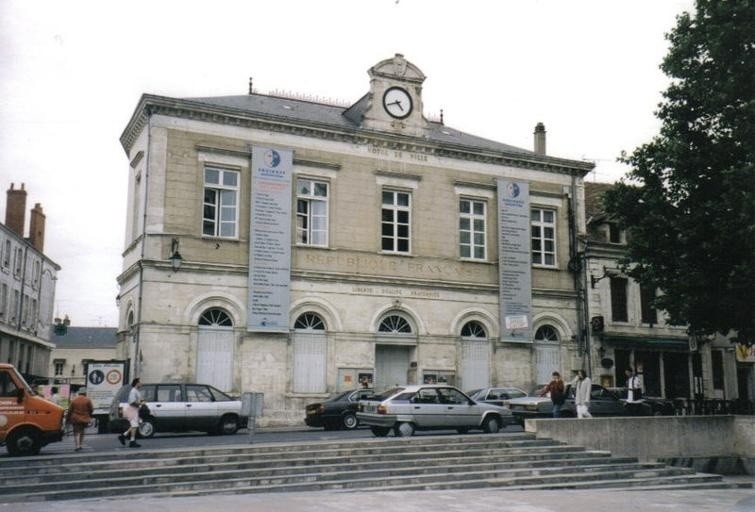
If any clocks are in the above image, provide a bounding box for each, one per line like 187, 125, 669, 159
383, 85, 414, 119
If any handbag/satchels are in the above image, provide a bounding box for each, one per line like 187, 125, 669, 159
633, 388, 642, 400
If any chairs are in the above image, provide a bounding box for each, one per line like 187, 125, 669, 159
670, 396, 755, 416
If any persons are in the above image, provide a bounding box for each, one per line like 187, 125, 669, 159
48, 386, 59, 404
539, 371, 564, 418
69, 387, 93, 451
118, 378, 145, 447
571, 370, 592, 417
624, 368, 641, 401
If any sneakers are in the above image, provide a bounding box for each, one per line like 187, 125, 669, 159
129, 441, 141, 447
118, 435, 126, 445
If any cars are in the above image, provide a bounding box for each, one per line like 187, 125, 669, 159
355, 383, 513, 437
305, 387, 380, 431
593, 386, 677, 417
457, 387, 530, 427
502, 382, 632, 428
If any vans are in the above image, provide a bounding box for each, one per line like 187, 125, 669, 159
0, 363, 65, 456
108, 381, 249, 436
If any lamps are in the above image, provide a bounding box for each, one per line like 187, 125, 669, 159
597, 346, 606, 360
589, 264, 620, 288
168, 238, 185, 273
